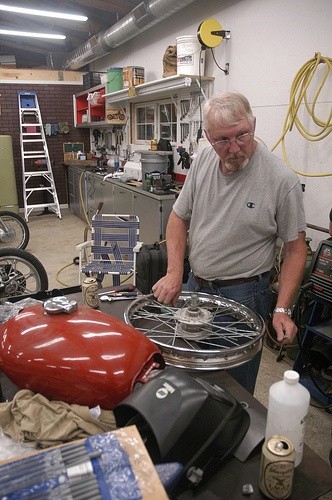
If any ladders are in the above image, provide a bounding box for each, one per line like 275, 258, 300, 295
18, 92, 61, 223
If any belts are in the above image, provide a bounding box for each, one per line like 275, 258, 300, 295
193, 271, 270, 288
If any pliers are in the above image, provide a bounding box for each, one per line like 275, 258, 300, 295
106, 292, 137, 297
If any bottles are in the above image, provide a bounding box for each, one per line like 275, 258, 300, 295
114, 154, 120, 167
147, 175, 152, 190
264, 370, 311, 467
80, 153, 86, 160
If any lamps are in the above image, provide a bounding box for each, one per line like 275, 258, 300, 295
0, 29, 67, 39
0, 5, 88, 22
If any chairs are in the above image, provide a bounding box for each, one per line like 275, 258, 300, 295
77, 202, 144, 287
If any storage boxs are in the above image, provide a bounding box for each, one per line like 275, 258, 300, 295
83, 71, 107, 90
63, 142, 97, 166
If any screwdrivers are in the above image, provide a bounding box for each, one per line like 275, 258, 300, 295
98, 284, 135, 295
277, 331, 288, 358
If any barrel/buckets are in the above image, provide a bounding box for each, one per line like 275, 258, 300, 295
122, 66, 144, 90
105, 66, 123, 94
176, 36, 207, 76
140, 153, 170, 190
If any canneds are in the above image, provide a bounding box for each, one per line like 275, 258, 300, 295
258, 435, 295, 500
151, 140, 157, 150
80, 153, 86, 160
82, 278, 99, 310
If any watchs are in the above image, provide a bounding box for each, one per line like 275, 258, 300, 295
274, 308, 292, 318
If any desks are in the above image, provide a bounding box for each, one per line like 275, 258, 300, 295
0, 284, 332, 500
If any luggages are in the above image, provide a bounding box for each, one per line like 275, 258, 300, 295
136, 241, 168, 295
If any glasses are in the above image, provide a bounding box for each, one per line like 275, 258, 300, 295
207, 125, 254, 149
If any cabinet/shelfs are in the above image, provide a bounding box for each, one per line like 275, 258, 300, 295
67, 165, 176, 245
73, 83, 127, 128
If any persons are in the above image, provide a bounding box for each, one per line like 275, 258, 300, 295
152, 91, 307, 396
